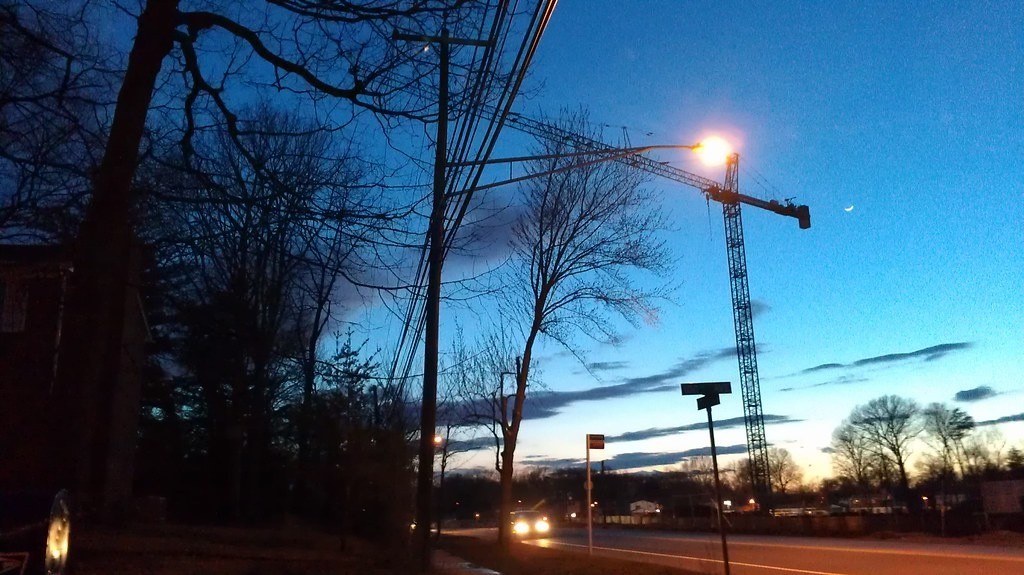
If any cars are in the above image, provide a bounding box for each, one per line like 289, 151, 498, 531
508, 509, 551, 539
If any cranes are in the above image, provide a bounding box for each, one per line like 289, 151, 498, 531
350, 58, 815, 539
